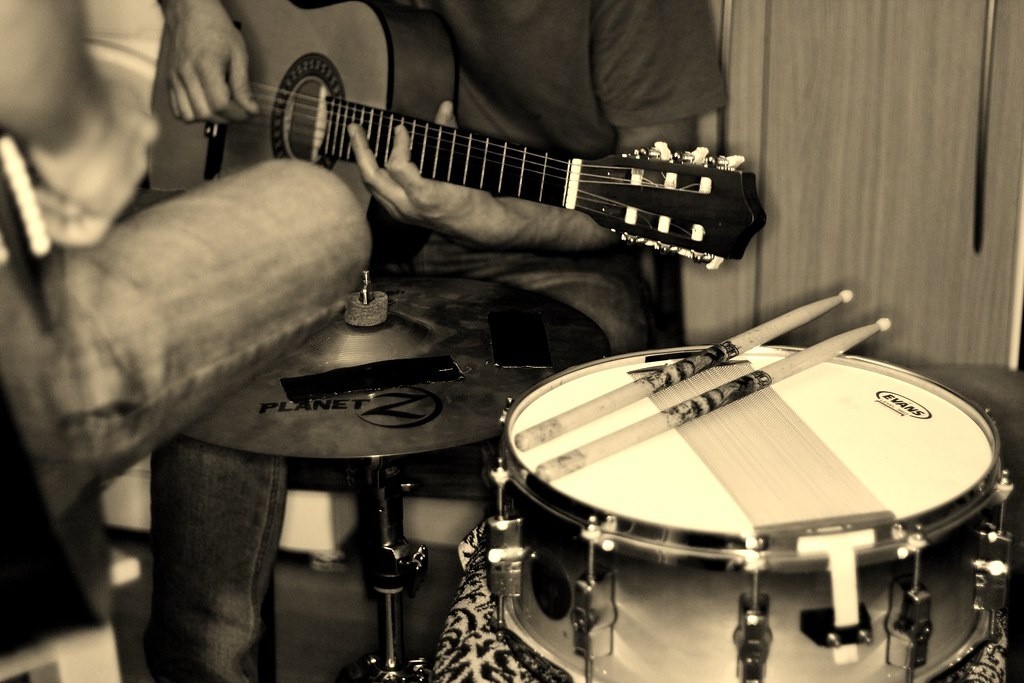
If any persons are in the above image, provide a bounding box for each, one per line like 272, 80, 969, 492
140, 0, 726, 683
1, 0, 370, 654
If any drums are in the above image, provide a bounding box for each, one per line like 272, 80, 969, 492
482, 337, 1013, 683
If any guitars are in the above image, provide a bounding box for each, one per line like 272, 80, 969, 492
0, 126, 113, 660
146, 0, 769, 273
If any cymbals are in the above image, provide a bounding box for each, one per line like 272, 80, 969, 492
176, 273, 615, 462
